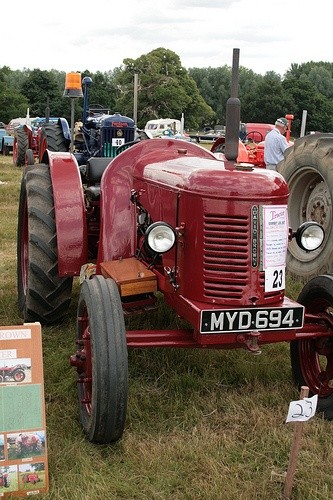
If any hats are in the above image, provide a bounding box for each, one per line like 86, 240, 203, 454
277, 118, 291, 133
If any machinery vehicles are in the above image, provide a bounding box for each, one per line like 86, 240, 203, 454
22, 473, 41, 485
1, 46, 333, 444
8, 432, 42, 458
0, 469, 10, 488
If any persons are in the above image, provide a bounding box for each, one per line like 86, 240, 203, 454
15, 434, 23, 456
263, 117, 289, 171
165, 124, 171, 136
75, 119, 83, 133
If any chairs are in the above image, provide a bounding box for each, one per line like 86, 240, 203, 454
86, 155, 118, 203
